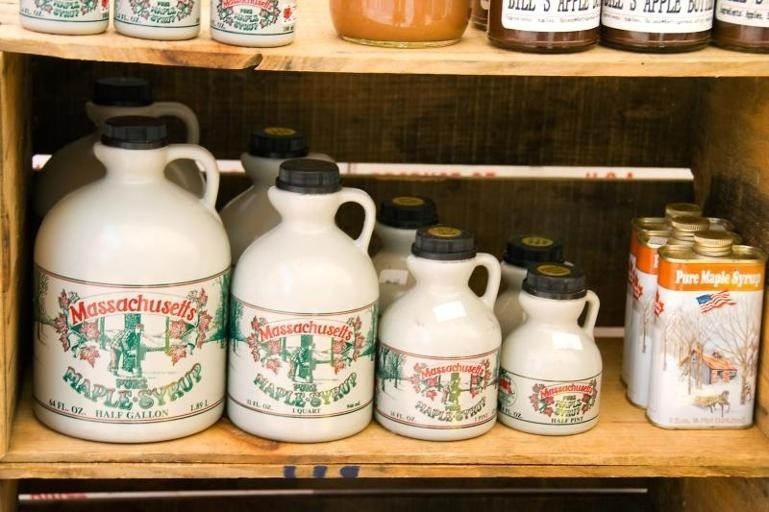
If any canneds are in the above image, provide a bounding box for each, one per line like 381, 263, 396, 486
471, 0, 769, 54
329, 0, 471, 49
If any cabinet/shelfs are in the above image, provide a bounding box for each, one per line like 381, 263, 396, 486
0, 0, 769, 510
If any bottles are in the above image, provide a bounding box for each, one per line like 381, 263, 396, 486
29, 116, 232, 447
493, 234, 576, 345
372, 196, 441, 319
372, 222, 504, 442
497, 262, 604, 437
218, 124, 337, 275
31, 73, 210, 235
224, 159, 382, 445
